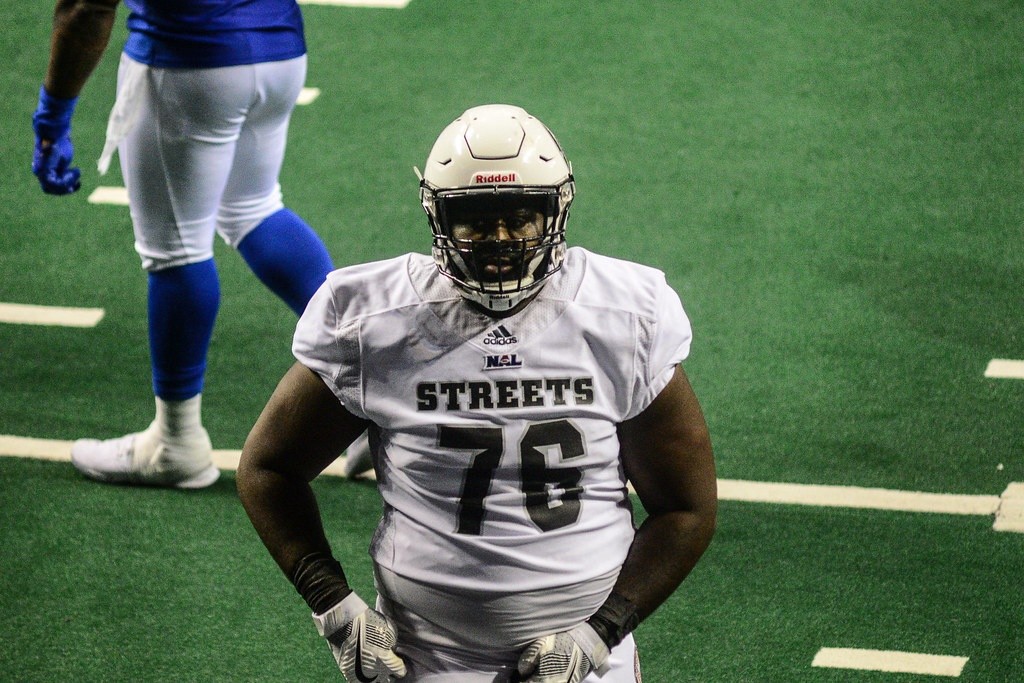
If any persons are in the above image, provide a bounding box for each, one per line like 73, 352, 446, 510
35, 0, 390, 488
240, 103, 718, 683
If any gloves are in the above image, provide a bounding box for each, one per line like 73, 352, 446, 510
30, 84, 82, 196
505, 621, 614, 683
311, 589, 408, 683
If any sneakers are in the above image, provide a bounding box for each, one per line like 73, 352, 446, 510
70, 422, 222, 489
344, 427, 373, 479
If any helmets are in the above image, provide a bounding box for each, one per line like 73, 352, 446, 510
413, 103, 577, 312
